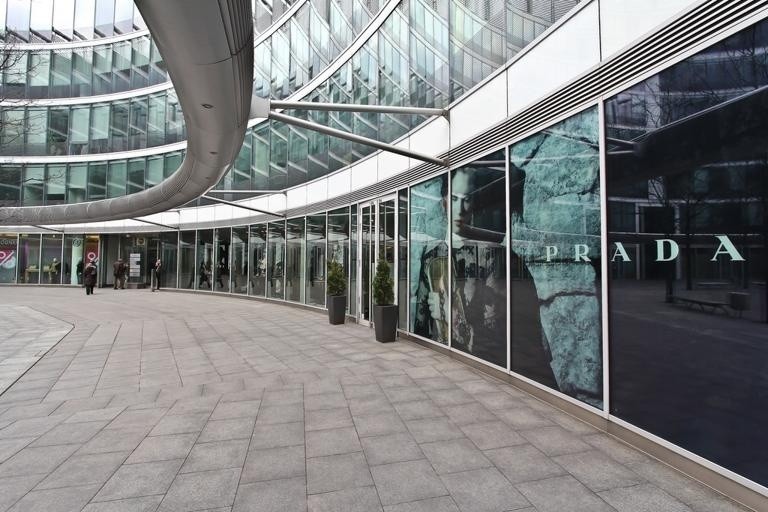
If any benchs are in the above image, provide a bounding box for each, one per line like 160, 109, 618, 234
672, 296, 731, 317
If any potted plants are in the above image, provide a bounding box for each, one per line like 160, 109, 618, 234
326, 261, 349, 325
371, 259, 399, 343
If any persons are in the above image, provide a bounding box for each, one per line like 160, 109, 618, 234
187, 244, 273, 289
49, 257, 84, 284
84, 260, 97, 295
414, 166, 561, 393
112, 259, 126, 289
